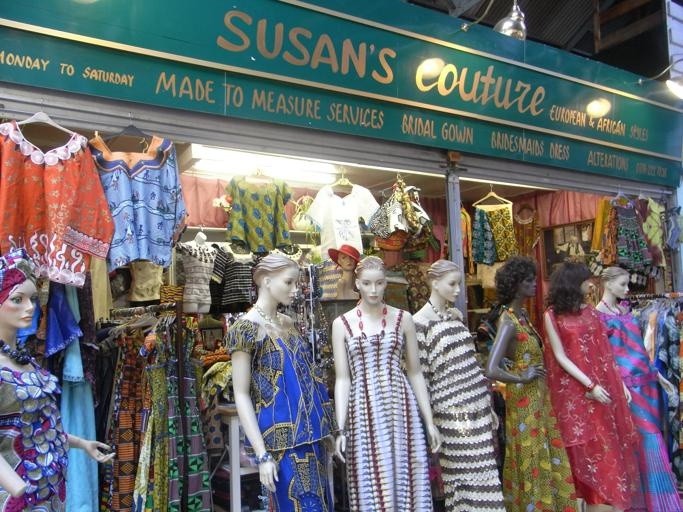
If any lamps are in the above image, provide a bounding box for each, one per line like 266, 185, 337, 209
459, 0, 527, 42
636, 60, 681, 99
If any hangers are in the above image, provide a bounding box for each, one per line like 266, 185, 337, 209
472, 182, 514, 208
87, 113, 173, 149
227, 162, 289, 188
607, 182, 682, 215
514, 204, 537, 217
0, 97, 87, 145
376, 171, 421, 194
320, 165, 369, 195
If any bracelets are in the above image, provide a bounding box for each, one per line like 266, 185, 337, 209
253, 451, 272, 465
588, 381, 598, 391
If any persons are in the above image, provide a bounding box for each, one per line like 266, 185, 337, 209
330, 255, 442, 512
329, 245, 359, 298
176, 232, 218, 312
227, 251, 335, 510
541, 262, 642, 512
413, 259, 505, 511
485, 254, 577, 512
214, 237, 259, 312
594, 265, 683, 511
0, 248, 115, 511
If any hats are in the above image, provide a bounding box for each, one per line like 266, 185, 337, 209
325, 244, 362, 270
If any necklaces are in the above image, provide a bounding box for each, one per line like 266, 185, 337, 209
252, 303, 283, 326
427, 299, 451, 322
506, 305, 534, 335
356, 300, 387, 345
600, 299, 623, 317
0, 336, 31, 366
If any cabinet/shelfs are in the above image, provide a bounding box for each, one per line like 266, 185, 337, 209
183, 222, 360, 303
